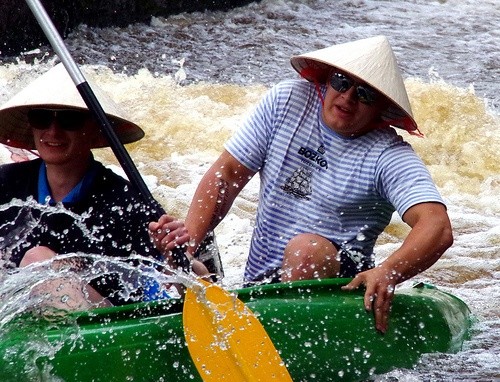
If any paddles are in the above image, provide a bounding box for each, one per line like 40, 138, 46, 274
26, 1, 294, 382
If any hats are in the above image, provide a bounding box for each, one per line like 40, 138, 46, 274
290, 34, 418, 133
0, 61, 144, 150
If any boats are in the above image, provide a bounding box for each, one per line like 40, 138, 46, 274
0, 276, 475, 382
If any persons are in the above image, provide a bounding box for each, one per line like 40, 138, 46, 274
184, 34, 454, 335
0, 61, 215, 318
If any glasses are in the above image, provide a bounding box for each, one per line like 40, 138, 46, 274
24, 105, 88, 132
328, 72, 380, 106
